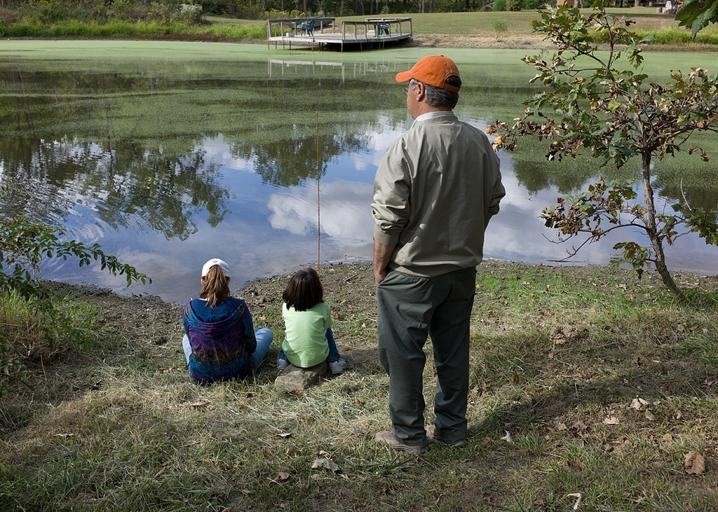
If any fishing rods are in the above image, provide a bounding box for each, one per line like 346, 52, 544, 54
316, 109, 321, 277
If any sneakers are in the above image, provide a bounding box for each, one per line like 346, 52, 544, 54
329, 360, 347, 375
375, 431, 430, 457
276, 352, 290, 372
424, 425, 466, 449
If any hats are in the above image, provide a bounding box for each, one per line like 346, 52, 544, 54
200, 257, 230, 279
395, 53, 462, 92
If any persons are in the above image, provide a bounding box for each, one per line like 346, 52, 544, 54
368, 53, 503, 454
278, 267, 346, 375
181, 257, 274, 383
